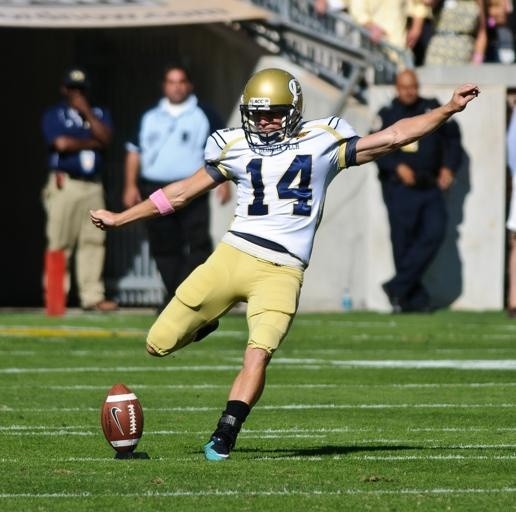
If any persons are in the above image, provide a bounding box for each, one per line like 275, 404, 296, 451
310, 0, 516, 65
85, 64, 482, 462
366, 68, 466, 316
501, 101, 516, 321
116, 65, 225, 317
35, 65, 120, 320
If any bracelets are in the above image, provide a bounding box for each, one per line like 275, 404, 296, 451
145, 189, 177, 221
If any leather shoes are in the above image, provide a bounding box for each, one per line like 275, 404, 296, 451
86, 300, 117, 311
382, 282, 403, 313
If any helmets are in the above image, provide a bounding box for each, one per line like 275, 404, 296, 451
239, 68, 304, 152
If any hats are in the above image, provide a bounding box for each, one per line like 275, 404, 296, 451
65, 66, 90, 89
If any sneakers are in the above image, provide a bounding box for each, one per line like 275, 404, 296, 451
193, 319, 219, 342
201, 432, 230, 461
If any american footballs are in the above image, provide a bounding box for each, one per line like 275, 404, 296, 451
100, 384, 143, 453
